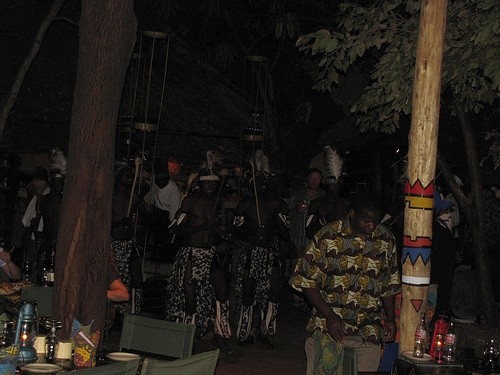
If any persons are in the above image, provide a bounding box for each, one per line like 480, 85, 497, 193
287, 187, 401, 375
0, 154, 500, 353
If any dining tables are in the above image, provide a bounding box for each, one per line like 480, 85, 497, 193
0, 333, 141, 375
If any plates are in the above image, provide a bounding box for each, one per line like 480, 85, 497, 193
22, 362, 64, 373
106, 352, 141, 361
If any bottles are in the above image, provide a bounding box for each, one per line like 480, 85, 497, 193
0, 301, 59, 353
442, 333, 456, 365
429, 315, 448, 357
447, 317, 455, 334
24, 249, 56, 288
434, 334, 444, 365
412, 312, 427, 358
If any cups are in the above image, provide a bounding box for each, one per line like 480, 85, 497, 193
0, 354, 18, 375
73, 339, 98, 368
56, 340, 72, 359
33, 335, 47, 354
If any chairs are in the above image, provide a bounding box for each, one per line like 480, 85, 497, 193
20, 287, 56, 330
139, 348, 220, 375
119, 315, 196, 358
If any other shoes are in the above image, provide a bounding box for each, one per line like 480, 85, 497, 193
212, 335, 231, 353
237, 334, 254, 345
256, 334, 274, 348
